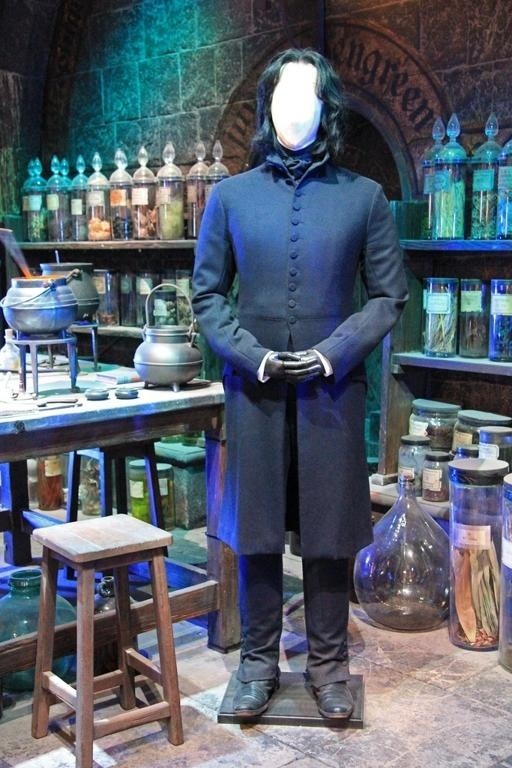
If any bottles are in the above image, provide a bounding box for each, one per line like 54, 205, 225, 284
353, 473, 448, 634
37, 456, 65, 510
397, 435, 433, 497
422, 277, 460, 359
0, 328, 21, 401
422, 157, 512, 241
459, 278, 491, 359
156, 462, 176, 532
421, 452, 450, 502
128, 459, 151, 524
91, 268, 194, 329
448, 459, 509, 649
499, 475, 512, 672
487, 278, 512, 362
22, 138, 230, 241
478, 426, 512, 473
451, 445, 479, 461
80, 456, 100, 515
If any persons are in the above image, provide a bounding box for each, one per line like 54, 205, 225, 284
192, 48, 409, 720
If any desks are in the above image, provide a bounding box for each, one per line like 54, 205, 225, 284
1, 354, 241, 674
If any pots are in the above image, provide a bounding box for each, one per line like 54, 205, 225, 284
2, 263, 100, 341
133, 283, 205, 392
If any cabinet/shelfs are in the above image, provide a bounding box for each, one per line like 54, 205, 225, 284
372, 200, 511, 485
0, 213, 230, 463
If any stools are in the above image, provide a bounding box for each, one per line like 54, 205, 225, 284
32, 513, 184, 768
